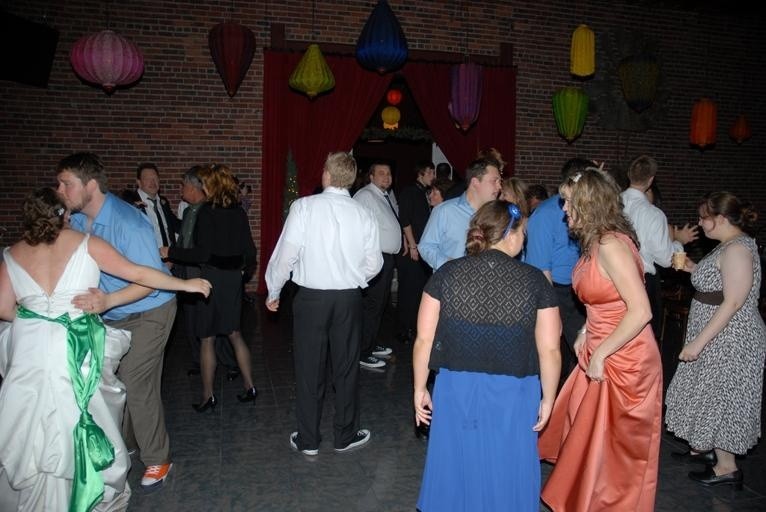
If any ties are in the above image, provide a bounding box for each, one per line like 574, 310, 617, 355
148, 197, 169, 248
385, 194, 403, 254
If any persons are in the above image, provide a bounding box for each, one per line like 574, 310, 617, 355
538, 166, 664, 511
352, 160, 409, 369
523, 184, 549, 218
524, 158, 603, 408
397, 161, 435, 345
170, 164, 240, 381
665, 189, 766, 485
264, 152, 384, 456
435, 162, 451, 179
159, 163, 258, 414
0, 186, 213, 512
498, 177, 529, 264
417, 154, 501, 444
615, 153, 701, 356
55, 149, 177, 488
412, 200, 562, 512
136, 162, 182, 377
428, 176, 455, 208
645, 181, 675, 242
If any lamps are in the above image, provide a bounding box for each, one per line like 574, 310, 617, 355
617, 0, 659, 114
386, 89, 402, 105
728, 1, 753, 144
690, 0, 717, 146
552, 1, 589, 146
381, 106, 401, 130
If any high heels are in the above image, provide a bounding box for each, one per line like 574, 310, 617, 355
237, 388, 257, 404
192, 395, 216, 412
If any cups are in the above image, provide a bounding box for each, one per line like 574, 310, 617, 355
671, 254, 688, 279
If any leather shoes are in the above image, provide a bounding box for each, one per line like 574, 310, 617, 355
228, 368, 239, 380
189, 368, 202, 376
689, 466, 744, 490
671, 450, 716, 465
413, 427, 429, 443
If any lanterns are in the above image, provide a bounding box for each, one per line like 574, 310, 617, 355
381, 107, 400, 125
387, 90, 401, 105
728, 122, 751, 142
552, 87, 589, 141
689, 97, 718, 147
618, 52, 659, 112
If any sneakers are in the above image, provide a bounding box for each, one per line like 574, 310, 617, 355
334, 429, 371, 451
140, 460, 172, 490
290, 432, 318, 455
359, 356, 385, 366
371, 346, 392, 356
